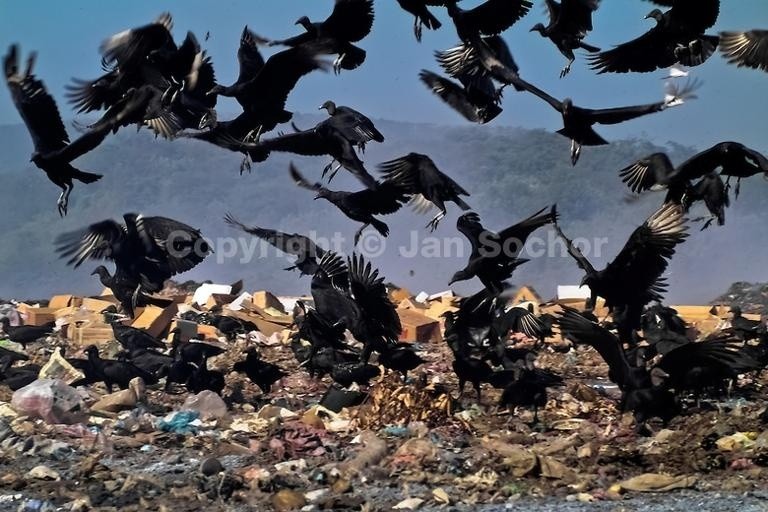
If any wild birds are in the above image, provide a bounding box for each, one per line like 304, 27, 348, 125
0, 0, 768, 430
